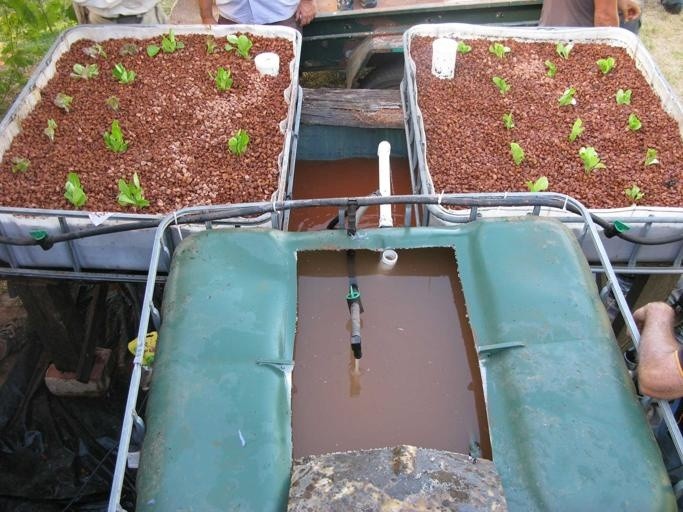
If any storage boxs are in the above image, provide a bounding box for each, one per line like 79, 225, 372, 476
400, 21, 683, 264
0, 21, 303, 271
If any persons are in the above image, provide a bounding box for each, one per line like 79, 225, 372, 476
198, 0, 317, 34
72, 0, 170, 25
626, 301, 682, 440
537, 0, 641, 34
336, 0, 376, 10
